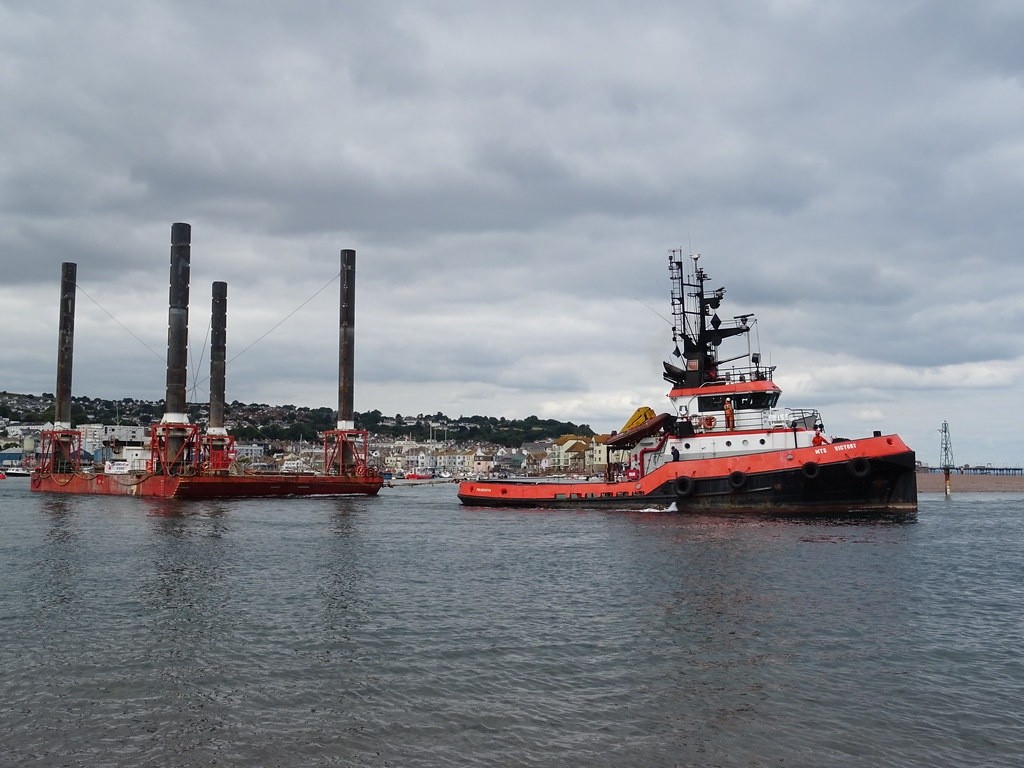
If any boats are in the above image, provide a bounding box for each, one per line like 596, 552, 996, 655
0, 472, 6, 479
564, 475, 586, 480
5, 467, 31, 477
456, 246, 921, 515
545, 474, 566, 478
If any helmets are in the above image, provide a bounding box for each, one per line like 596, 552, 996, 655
816, 432, 820, 434
725, 398, 730, 401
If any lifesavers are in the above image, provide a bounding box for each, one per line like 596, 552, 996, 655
145, 459, 154, 474
202, 460, 213, 473
728, 470, 748, 490
688, 414, 703, 430
672, 475, 696, 499
702, 416, 717, 431
356, 466, 366, 475
846, 456, 873, 483
800, 461, 822, 480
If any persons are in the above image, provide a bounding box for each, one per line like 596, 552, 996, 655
724, 397, 734, 432
812, 431, 830, 446
671, 446, 680, 462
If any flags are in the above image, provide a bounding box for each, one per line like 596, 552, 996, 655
673, 346, 681, 358
